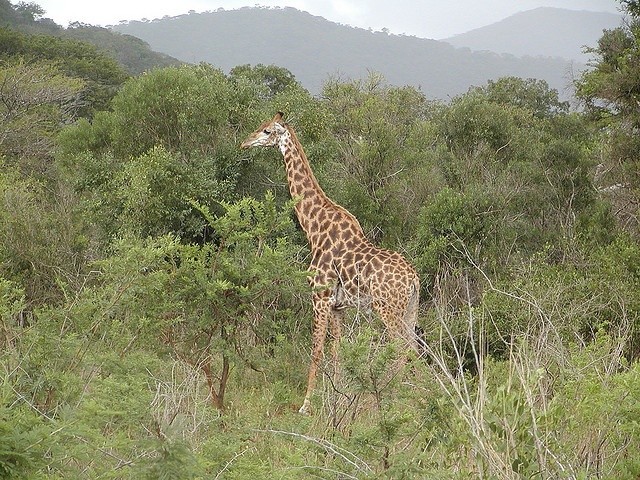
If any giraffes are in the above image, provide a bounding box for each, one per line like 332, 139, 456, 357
239, 112, 420, 417
